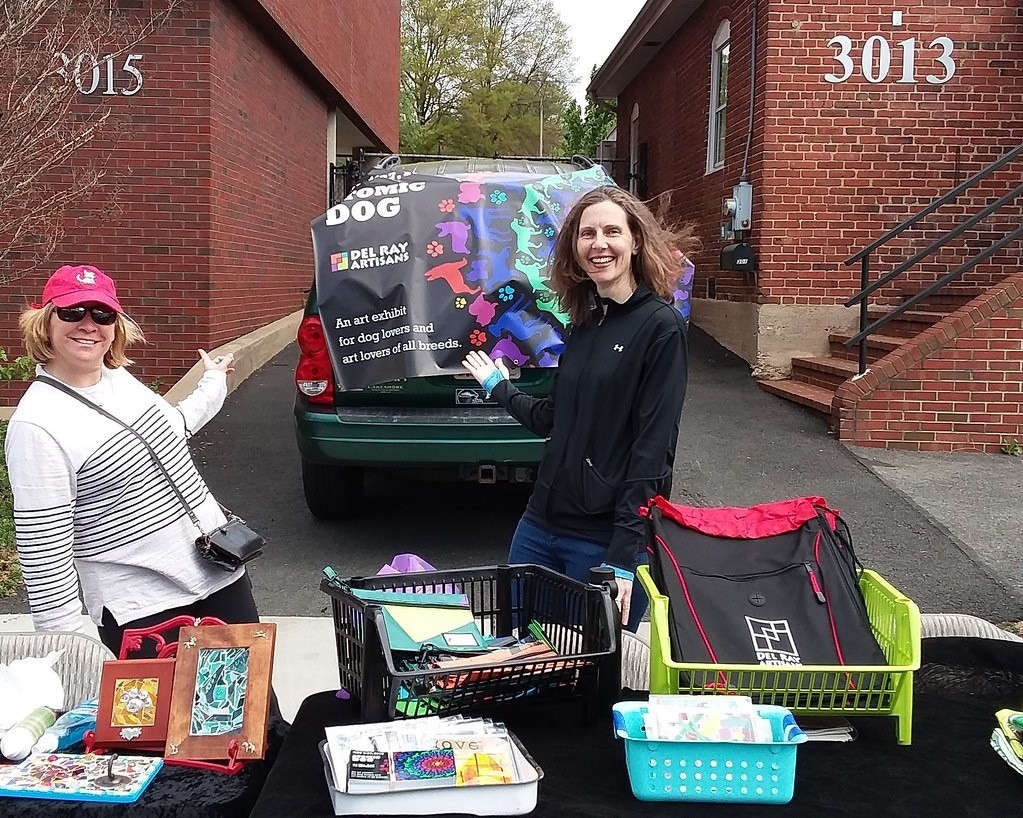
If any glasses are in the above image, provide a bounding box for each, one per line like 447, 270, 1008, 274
52, 306, 118, 325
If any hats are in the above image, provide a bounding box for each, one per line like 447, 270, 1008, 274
31, 265, 124, 315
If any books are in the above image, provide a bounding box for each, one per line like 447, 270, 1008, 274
324, 713, 523, 796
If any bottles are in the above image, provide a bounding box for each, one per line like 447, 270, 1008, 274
1, 706, 56, 760
584, 566, 620, 704
32, 700, 99, 753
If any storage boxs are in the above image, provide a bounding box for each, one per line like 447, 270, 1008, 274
317, 563, 616, 722
636, 564, 918, 746
612, 701, 807, 803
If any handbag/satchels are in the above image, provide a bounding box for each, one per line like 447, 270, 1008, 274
638, 496, 893, 714
195, 519, 268, 574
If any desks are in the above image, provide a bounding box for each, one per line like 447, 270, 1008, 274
250, 614, 1023, 818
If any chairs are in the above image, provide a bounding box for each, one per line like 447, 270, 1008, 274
0, 630, 118, 712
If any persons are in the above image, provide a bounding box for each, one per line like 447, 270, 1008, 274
4, 264, 260, 663
463, 184, 692, 628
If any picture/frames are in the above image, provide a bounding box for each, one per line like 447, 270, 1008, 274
95, 658, 176, 748
164, 623, 278, 761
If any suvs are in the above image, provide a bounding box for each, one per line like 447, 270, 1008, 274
292, 153, 623, 519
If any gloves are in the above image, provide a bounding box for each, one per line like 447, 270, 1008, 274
460, 348, 510, 395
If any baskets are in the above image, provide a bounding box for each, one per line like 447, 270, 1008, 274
612, 700, 810, 803
320, 564, 620, 729
638, 556, 924, 747
